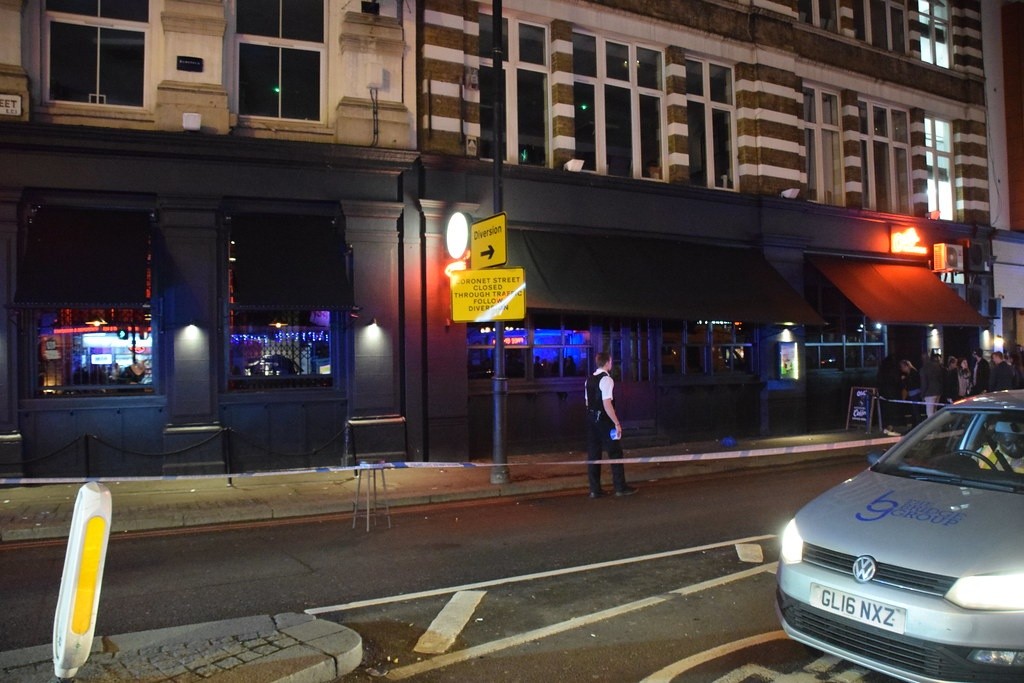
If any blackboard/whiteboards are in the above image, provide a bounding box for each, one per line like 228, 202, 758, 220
846, 386, 876, 428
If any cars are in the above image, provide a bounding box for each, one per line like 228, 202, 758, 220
776, 390, 1024, 683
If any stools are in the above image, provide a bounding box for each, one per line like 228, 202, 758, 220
352, 466, 392, 532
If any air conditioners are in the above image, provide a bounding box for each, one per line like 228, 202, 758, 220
933, 243, 963, 270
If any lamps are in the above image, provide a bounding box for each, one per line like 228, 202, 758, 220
925, 212, 940, 220
780, 188, 800, 199
563, 158, 584, 173
182, 112, 202, 132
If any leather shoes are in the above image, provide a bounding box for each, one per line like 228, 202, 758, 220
589, 488, 615, 499
616, 484, 639, 496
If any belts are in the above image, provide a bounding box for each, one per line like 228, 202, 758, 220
588, 409, 607, 415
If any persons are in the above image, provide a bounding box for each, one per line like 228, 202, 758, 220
972, 421, 1024, 474
584, 353, 638, 498
879, 344, 1024, 436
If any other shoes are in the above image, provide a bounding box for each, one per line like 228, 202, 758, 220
883, 428, 901, 436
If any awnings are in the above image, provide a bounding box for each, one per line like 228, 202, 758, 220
813, 263, 993, 328
